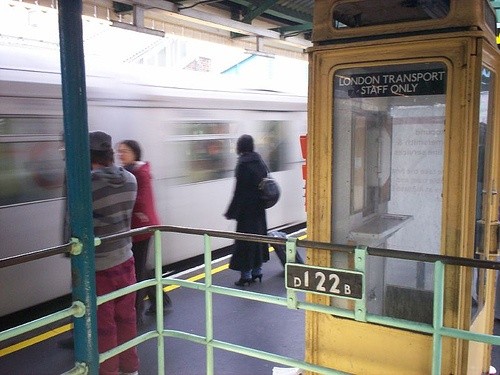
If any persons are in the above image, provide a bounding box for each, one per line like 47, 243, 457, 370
473, 122, 500, 300
57, 131, 139, 375
225, 135, 269, 286
118, 139, 172, 322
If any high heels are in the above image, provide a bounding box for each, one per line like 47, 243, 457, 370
234, 277, 253, 287
252, 272, 263, 283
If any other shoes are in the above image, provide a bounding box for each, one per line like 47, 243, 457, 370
137, 302, 174, 328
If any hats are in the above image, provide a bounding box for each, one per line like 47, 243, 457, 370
89, 130, 113, 151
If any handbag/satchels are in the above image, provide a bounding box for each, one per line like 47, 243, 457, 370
260, 177, 281, 209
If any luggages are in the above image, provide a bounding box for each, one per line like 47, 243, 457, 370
268, 231, 304, 268
